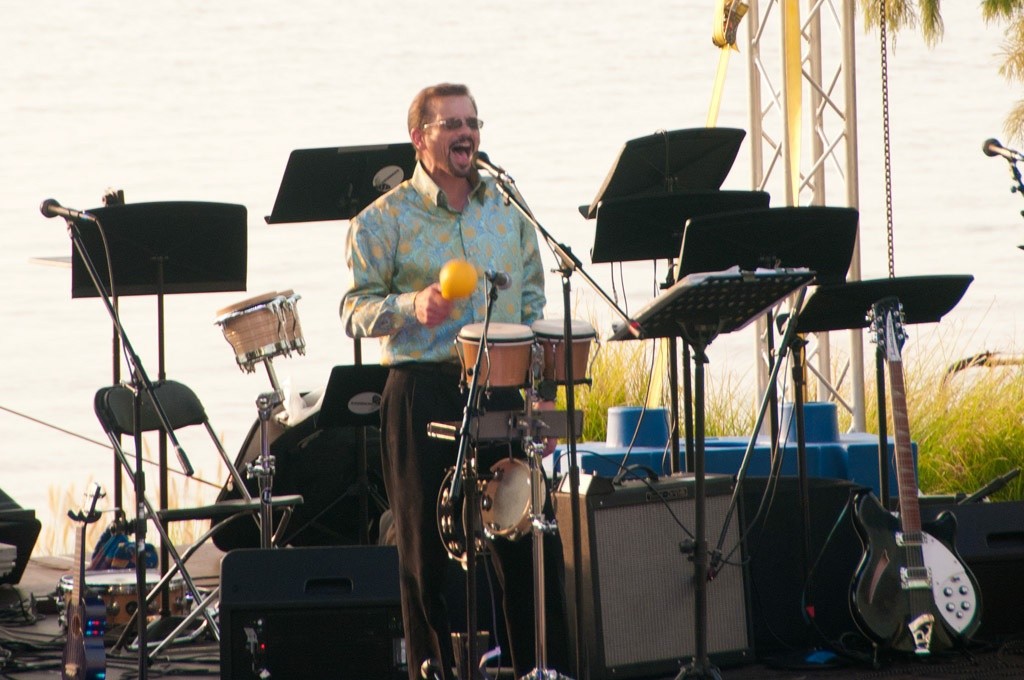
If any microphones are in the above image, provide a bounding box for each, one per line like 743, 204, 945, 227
470, 151, 514, 184
484, 270, 512, 290
982, 138, 1024, 162
40, 198, 96, 222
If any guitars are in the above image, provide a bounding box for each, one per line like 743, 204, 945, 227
60, 480, 108, 679
846, 294, 984, 658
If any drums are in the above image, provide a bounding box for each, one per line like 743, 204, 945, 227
214, 287, 292, 369
479, 457, 546, 540
457, 321, 533, 392
275, 289, 304, 355
530, 317, 595, 384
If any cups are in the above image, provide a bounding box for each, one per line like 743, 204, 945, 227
452, 632, 490, 680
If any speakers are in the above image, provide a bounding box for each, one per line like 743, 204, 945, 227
218, 546, 400, 680
549, 472, 754, 680
921, 500, 1024, 633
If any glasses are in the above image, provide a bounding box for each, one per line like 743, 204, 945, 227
424, 118, 484, 130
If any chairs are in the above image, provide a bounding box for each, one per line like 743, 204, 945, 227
94, 378, 304, 669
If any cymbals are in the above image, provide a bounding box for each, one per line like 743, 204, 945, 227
483, 454, 548, 542
434, 466, 491, 564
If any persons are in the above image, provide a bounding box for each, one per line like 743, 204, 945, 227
338, 85, 545, 680
